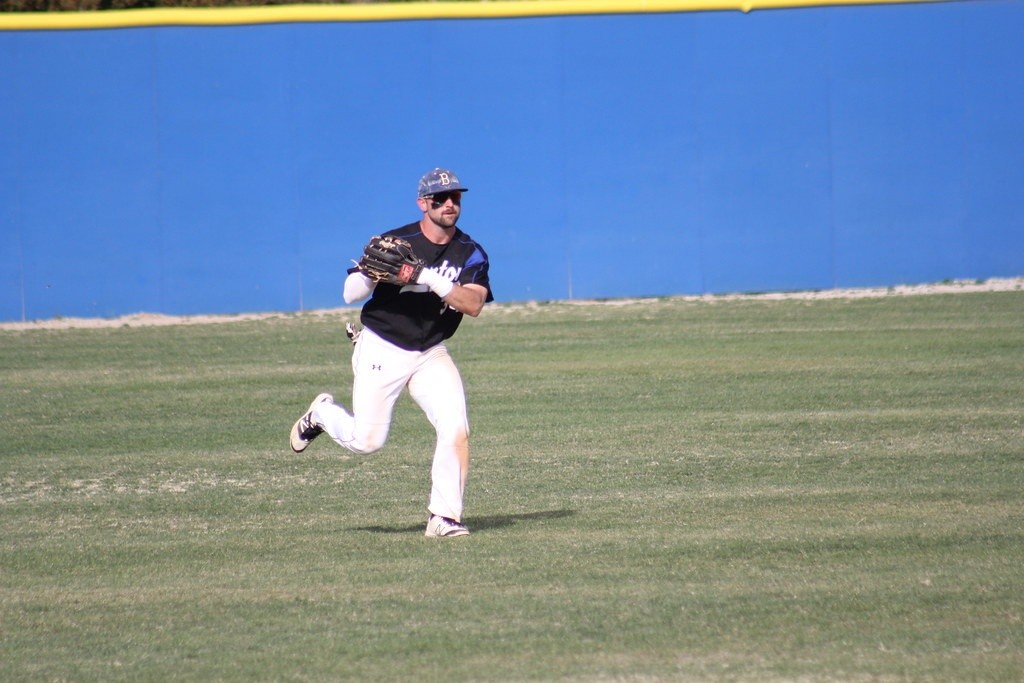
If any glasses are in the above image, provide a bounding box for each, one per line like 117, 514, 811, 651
422, 190, 461, 203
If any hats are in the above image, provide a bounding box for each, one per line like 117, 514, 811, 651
419, 168, 468, 197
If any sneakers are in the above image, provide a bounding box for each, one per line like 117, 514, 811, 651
289, 392, 338, 452
425, 514, 468, 536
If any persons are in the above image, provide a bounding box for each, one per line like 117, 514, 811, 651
289, 168, 494, 539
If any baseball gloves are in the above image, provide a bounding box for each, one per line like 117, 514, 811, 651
358, 235, 424, 286
